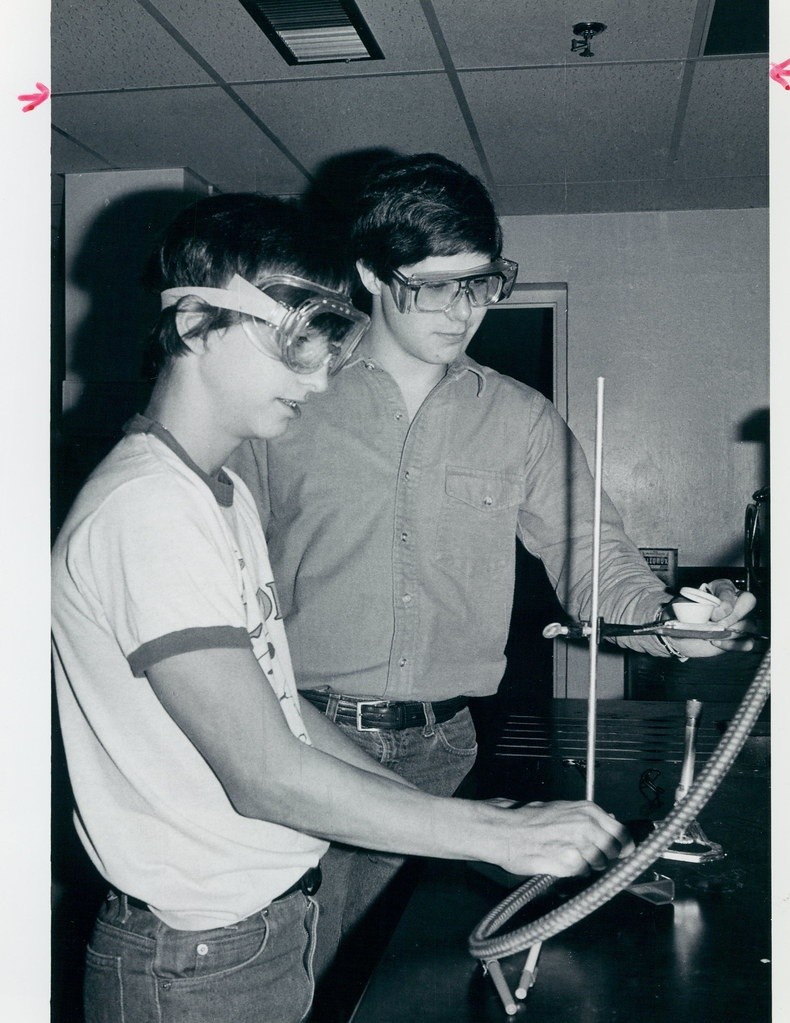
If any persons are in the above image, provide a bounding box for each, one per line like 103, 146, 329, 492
225, 154, 756, 1023
51, 192, 634, 1023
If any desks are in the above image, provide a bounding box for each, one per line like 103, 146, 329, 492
348, 756, 772, 1023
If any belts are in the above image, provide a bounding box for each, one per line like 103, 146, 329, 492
298, 691, 467, 732
126, 866, 323, 911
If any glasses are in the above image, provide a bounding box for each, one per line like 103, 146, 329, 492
386, 257, 518, 313
229, 276, 372, 375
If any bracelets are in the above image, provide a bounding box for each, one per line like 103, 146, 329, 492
656, 607, 688, 663
